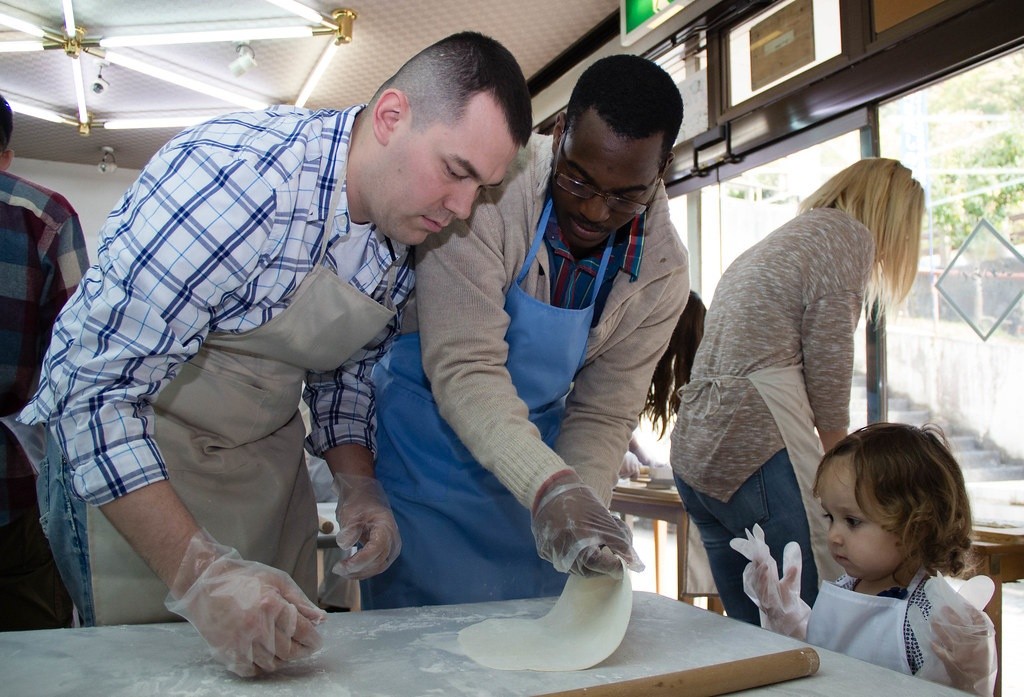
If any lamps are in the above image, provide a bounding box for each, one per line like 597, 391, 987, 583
97, 147, 119, 177
1, 1, 358, 137
227, 44, 258, 76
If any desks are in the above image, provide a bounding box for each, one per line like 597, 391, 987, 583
610, 474, 695, 608
705, 520, 1024, 696
2, 588, 982, 697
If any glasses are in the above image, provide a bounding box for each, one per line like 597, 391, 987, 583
552, 119, 667, 215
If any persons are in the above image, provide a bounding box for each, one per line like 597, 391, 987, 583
617, 288, 707, 481
750, 420, 999, 697
357, 52, 690, 609
16, 30, 532, 687
672, 156, 927, 628
0, 90, 90, 632
